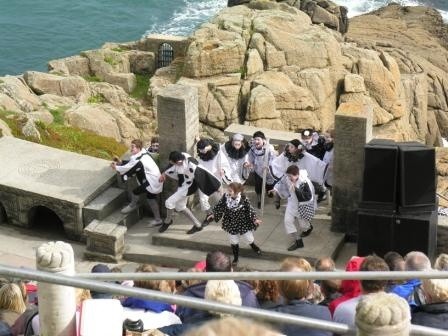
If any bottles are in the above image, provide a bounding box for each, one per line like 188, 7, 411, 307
122, 314, 144, 336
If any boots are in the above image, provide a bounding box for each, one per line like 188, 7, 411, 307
231, 243, 240, 265
249, 241, 262, 256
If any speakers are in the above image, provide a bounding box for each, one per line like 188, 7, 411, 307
356, 139, 439, 269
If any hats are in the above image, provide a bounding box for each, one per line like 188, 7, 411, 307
89, 264, 113, 301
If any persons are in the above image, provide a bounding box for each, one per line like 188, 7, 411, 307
0, 128, 448, 336
207, 182, 263, 265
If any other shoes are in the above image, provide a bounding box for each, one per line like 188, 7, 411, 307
187, 224, 203, 235
300, 222, 314, 237
158, 218, 174, 233
121, 204, 138, 214
202, 215, 213, 227
149, 219, 162, 226
287, 239, 304, 251
